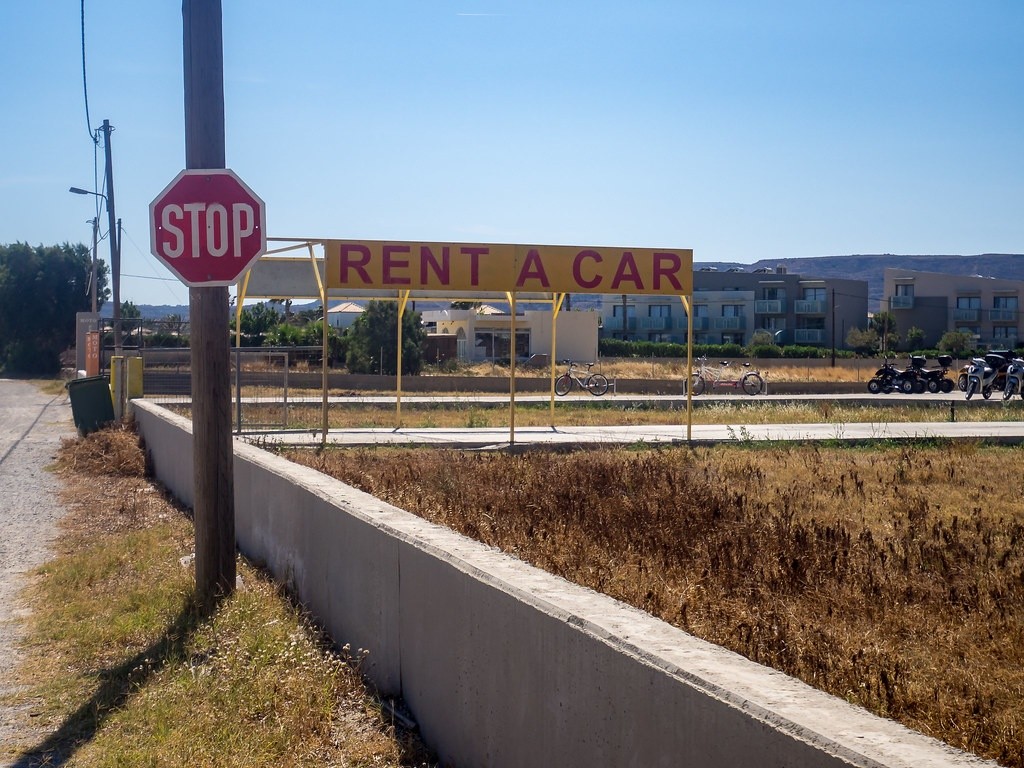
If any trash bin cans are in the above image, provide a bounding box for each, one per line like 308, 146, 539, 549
65, 374, 115, 437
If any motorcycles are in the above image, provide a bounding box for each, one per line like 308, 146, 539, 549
867, 354, 954, 394
957, 349, 1024, 400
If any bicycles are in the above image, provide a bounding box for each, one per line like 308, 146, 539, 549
555, 359, 608, 396
685, 354, 763, 397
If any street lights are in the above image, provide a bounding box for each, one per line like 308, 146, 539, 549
69, 187, 123, 421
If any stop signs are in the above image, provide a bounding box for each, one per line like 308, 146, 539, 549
148, 169, 267, 287
